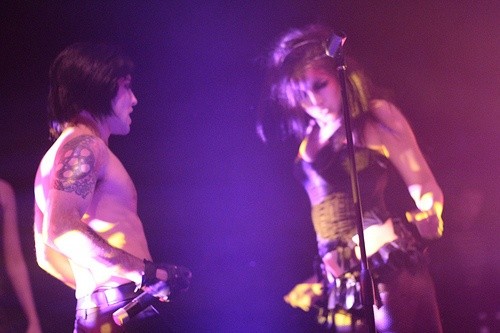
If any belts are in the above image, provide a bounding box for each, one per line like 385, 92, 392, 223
76, 281, 142, 310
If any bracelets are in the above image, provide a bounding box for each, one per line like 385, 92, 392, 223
135, 258, 157, 293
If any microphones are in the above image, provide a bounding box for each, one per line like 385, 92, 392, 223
112, 280, 171, 325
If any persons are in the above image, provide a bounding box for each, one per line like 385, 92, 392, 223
32, 42, 192, 333
258, 24, 444, 333
0, 179, 41, 333
